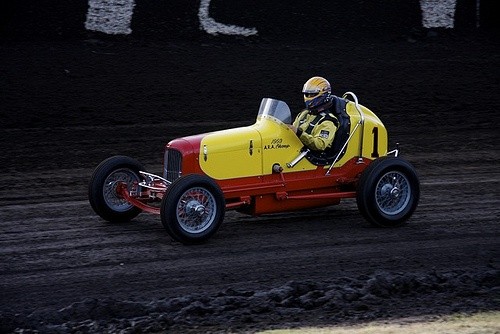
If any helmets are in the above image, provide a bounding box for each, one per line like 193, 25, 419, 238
301, 76, 331, 110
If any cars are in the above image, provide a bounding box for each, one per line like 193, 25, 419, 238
87, 92, 421, 246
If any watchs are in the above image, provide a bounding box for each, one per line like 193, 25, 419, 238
296, 128, 303, 138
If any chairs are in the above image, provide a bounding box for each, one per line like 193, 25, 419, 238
304, 94, 350, 165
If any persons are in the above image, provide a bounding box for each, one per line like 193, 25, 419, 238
285, 76, 340, 151
